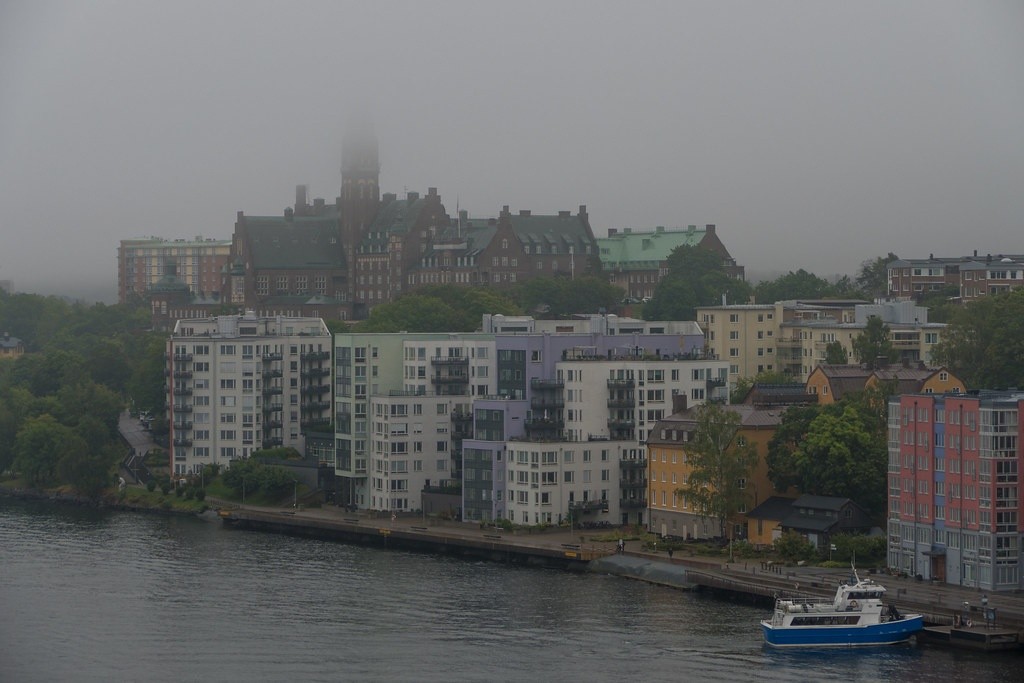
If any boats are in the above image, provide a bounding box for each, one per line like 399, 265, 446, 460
758, 560, 927, 648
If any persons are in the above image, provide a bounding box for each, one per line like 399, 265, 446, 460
981, 594, 989, 621
668, 547, 673, 561
617, 537, 625, 555
